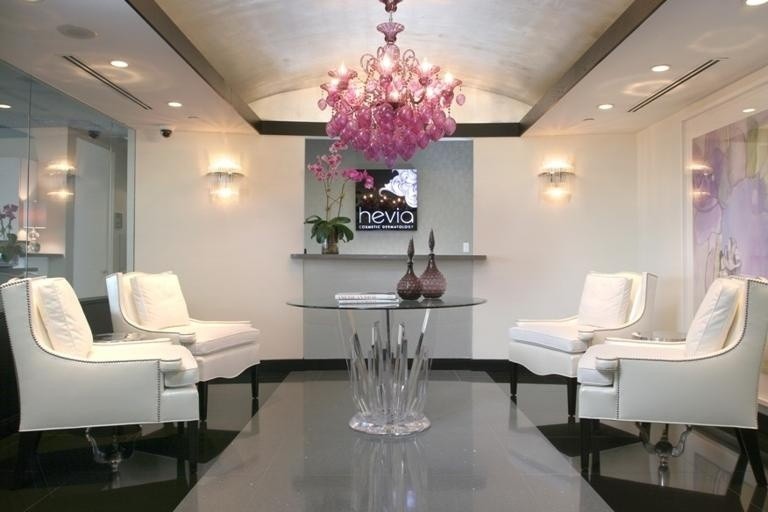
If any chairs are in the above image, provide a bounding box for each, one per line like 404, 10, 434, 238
576, 277, 767, 488
106, 272, 262, 423
1, 275, 199, 474
505, 270, 659, 425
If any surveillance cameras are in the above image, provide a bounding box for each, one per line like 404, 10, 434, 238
161, 128, 174, 137
88, 130, 100, 139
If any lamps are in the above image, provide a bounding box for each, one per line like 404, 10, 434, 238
317, 0, 466, 168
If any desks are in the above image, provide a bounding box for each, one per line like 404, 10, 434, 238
286, 296, 488, 438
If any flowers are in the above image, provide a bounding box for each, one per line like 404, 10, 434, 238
0, 203, 19, 240
307, 154, 374, 221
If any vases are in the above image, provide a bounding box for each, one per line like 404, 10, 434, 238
0, 241, 26, 267
304, 216, 354, 254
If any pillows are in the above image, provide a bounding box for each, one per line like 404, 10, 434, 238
128, 270, 189, 328
26, 278, 93, 359
578, 272, 633, 328
684, 277, 745, 356
